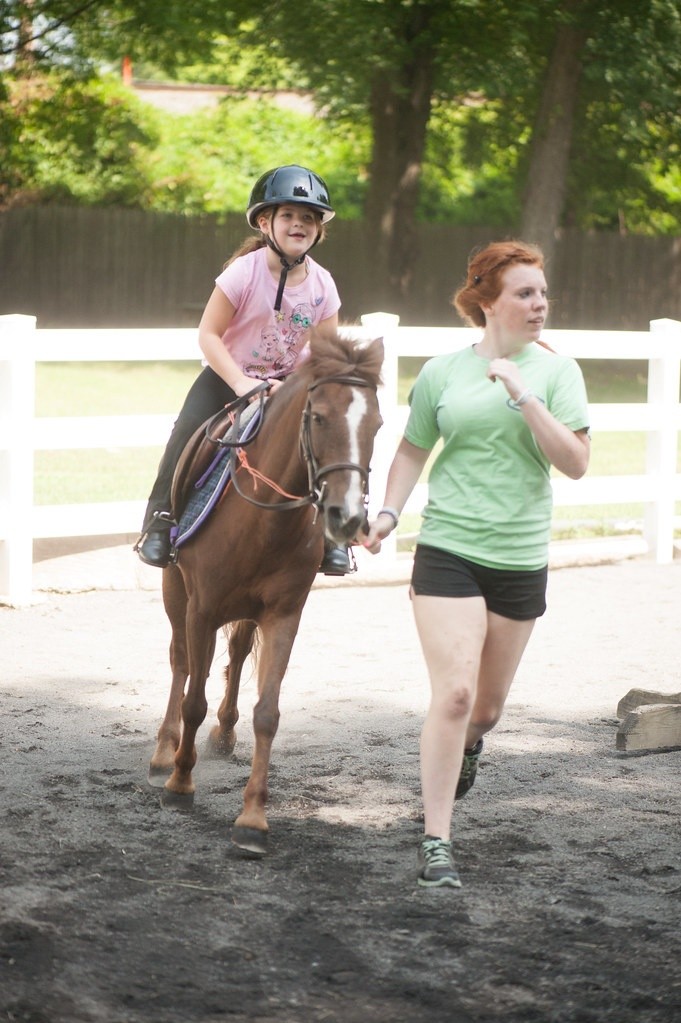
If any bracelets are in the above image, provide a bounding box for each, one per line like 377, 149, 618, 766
515, 387, 532, 410
378, 507, 400, 532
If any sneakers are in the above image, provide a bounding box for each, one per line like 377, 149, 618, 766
414, 834, 462, 888
454, 738, 483, 800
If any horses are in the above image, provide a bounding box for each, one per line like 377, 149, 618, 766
147, 322, 385, 859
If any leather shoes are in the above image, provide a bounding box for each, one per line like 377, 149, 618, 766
318, 541, 349, 574
139, 526, 172, 568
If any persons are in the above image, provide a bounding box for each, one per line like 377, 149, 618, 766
137, 165, 353, 575
364, 239, 591, 885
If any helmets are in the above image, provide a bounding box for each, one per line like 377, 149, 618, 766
246, 165, 335, 233
